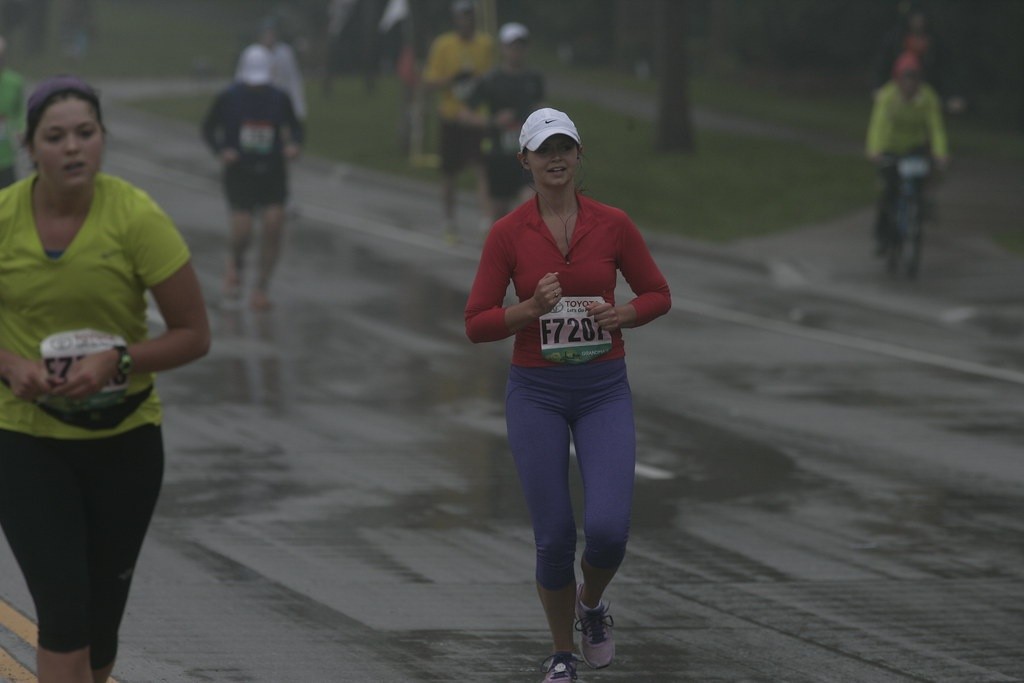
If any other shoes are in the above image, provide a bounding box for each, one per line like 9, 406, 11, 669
220, 264, 275, 317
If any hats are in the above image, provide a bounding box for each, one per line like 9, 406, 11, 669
519, 107, 583, 152
893, 51, 922, 73
234, 43, 278, 85
499, 21, 530, 45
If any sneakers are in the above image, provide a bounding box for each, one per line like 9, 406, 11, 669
541, 651, 578, 683
575, 582, 617, 670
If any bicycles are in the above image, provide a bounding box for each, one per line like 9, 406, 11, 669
875, 145, 936, 278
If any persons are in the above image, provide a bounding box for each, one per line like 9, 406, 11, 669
203, 40, 307, 310
2, 49, 26, 193
860, 51, 950, 277
0, 72, 212, 682
467, 21, 555, 222
236, 16, 306, 124
459, 105, 671, 683
421, 2, 495, 217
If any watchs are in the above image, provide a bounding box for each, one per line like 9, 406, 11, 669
113, 345, 134, 385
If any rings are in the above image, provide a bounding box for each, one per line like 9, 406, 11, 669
554, 292, 559, 297
